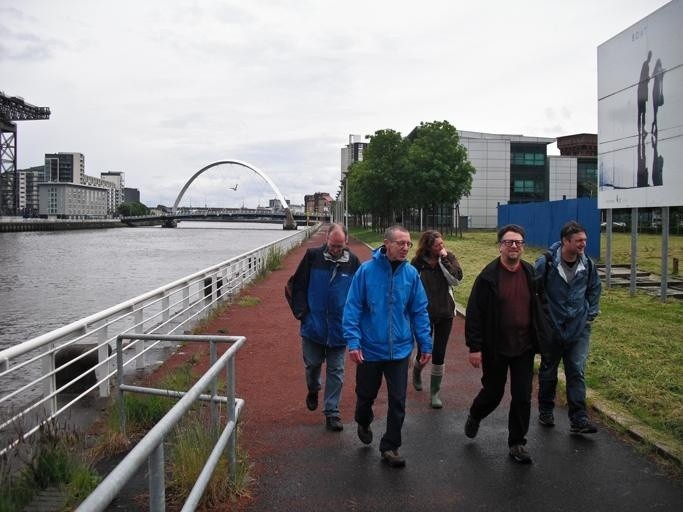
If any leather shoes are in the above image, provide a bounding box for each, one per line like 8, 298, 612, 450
326, 416, 343, 430
307, 390, 318, 410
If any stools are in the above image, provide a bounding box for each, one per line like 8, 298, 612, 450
203, 277, 225, 299
247, 254, 264, 274
54, 343, 112, 396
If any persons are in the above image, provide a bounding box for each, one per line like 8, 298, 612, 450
637, 50, 653, 135
534, 219, 601, 434
650, 57, 667, 135
650, 136, 664, 186
342, 224, 433, 468
637, 136, 647, 187
464, 225, 550, 464
408, 230, 463, 409
283, 223, 361, 432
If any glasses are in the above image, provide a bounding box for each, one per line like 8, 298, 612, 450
500, 239, 526, 247
392, 239, 412, 249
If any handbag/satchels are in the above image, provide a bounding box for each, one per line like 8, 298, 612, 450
285, 247, 311, 320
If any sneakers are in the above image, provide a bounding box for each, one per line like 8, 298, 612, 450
510, 440, 532, 462
570, 415, 598, 436
381, 449, 405, 468
539, 409, 556, 426
464, 412, 479, 439
358, 421, 373, 444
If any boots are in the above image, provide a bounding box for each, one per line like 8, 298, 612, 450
430, 374, 443, 409
413, 364, 423, 390
